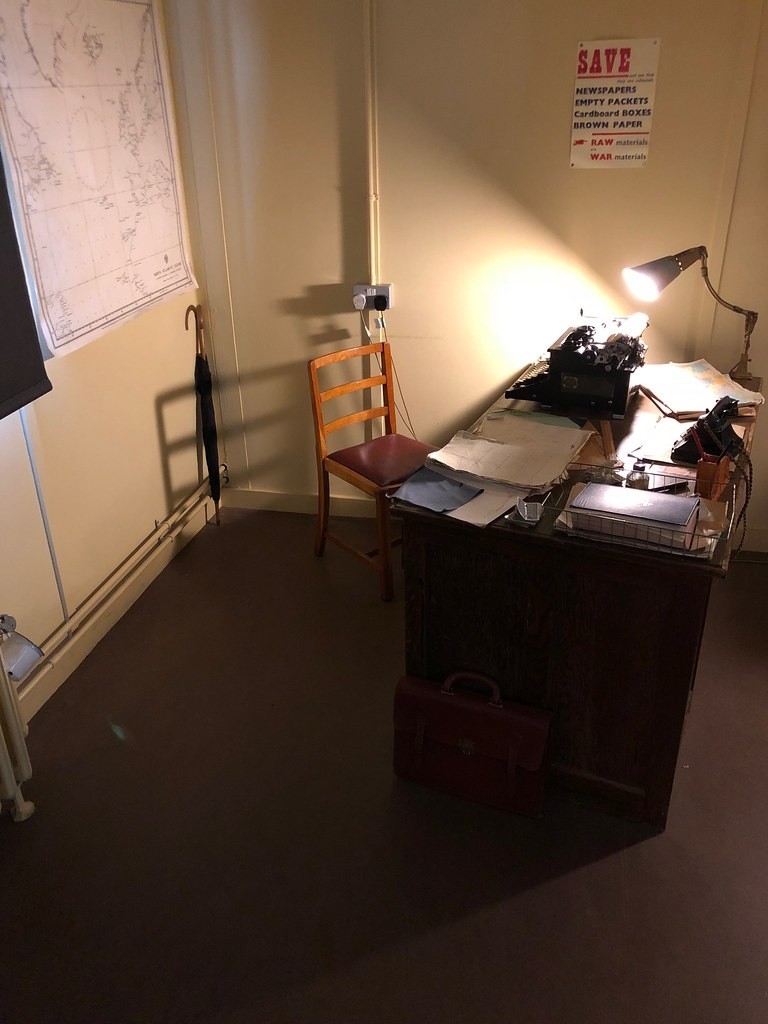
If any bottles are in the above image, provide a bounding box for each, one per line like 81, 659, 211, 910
627, 463, 650, 489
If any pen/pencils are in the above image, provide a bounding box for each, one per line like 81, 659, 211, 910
650, 480, 689, 494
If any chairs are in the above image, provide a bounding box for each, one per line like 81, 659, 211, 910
307, 341, 441, 601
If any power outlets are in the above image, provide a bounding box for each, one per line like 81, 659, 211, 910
352, 283, 393, 309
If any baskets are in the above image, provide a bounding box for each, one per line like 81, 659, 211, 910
540, 461, 736, 568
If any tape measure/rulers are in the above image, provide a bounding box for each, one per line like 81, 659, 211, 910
601, 419, 618, 463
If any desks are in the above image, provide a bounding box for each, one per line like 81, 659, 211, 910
391, 358, 763, 828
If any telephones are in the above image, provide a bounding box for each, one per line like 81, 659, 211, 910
670, 396, 744, 465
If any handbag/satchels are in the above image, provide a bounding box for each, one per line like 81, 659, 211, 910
671, 397, 754, 563
392, 670, 557, 820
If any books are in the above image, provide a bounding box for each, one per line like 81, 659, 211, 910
553, 478, 729, 561
628, 358, 765, 472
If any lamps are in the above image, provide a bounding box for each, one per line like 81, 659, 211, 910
621, 244, 758, 380
